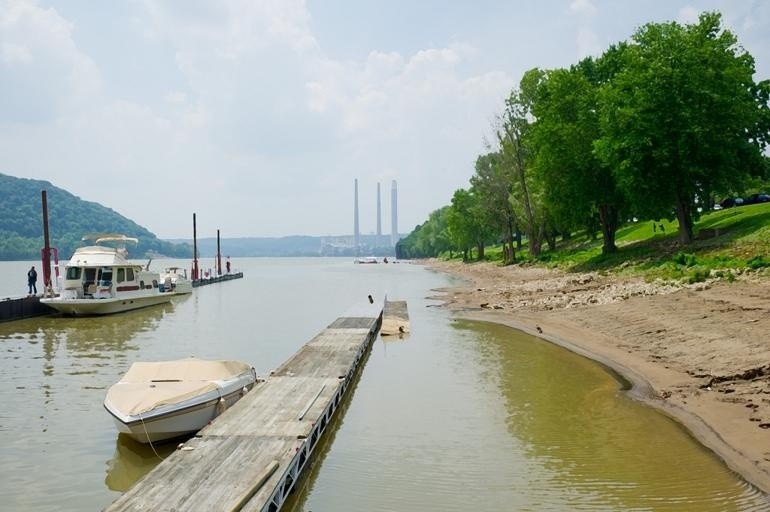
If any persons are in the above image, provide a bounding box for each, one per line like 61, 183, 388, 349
27, 266, 37, 294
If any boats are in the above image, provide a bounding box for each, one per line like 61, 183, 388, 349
103, 355, 261, 447
39, 233, 193, 317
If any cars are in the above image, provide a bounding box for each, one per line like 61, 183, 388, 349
713, 194, 770, 210
